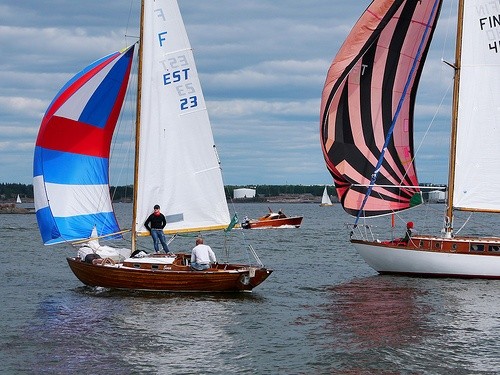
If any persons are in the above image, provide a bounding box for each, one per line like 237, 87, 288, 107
190, 239, 217, 270
278, 211, 287, 218
144, 204, 170, 254
401, 226, 411, 243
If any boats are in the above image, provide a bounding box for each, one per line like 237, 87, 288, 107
240, 207, 303, 230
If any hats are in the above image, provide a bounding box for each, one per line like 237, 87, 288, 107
154, 205, 160, 209
278, 211, 282, 214
407, 222, 413, 228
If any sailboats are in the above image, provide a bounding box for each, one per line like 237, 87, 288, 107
31, 0, 275, 294
16, 194, 22, 204
314, 0, 500, 281
318, 186, 336, 207
